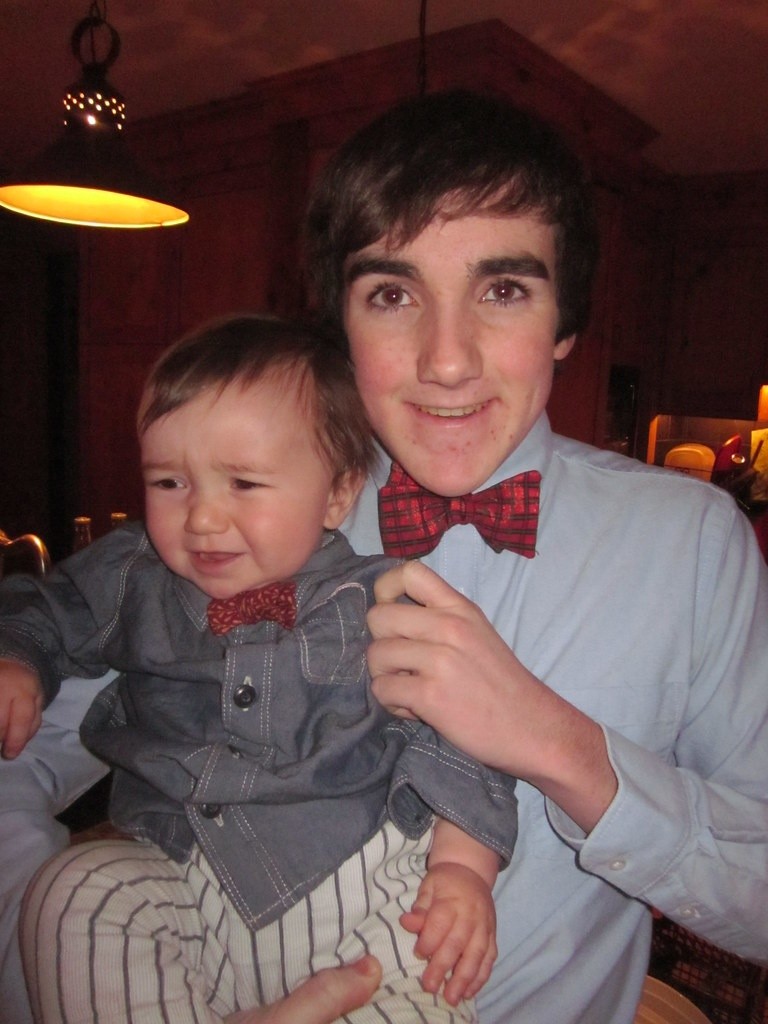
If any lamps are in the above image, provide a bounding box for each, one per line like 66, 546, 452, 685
1, 0, 190, 228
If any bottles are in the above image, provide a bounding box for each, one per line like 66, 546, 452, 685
111, 513, 128, 531
72, 517, 94, 556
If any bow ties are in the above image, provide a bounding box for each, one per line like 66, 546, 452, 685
377, 460, 540, 562
205, 577, 300, 637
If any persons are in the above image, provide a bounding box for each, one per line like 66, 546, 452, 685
0, 92, 768, 1024
0, 312, 519, 1024
